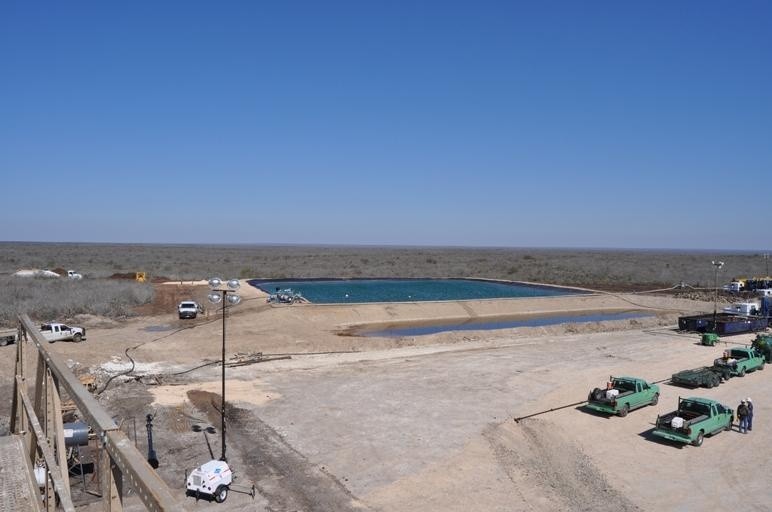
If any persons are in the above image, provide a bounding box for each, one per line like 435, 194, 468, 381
735, 399, 749, 433
745, 397, 753, 431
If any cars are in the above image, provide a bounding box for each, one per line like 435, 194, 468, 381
66, 270, 81, 280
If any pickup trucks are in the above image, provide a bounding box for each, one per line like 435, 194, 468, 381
650, 394, 735, 446
14, 323, 86, 344
585, 375, 659, 417
178, 300, 198, 318
714, 348, 764, 376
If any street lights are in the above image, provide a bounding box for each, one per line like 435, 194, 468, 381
207, 277, 241, 463
712, 261, 724, 327
190, 422, 216, 461
